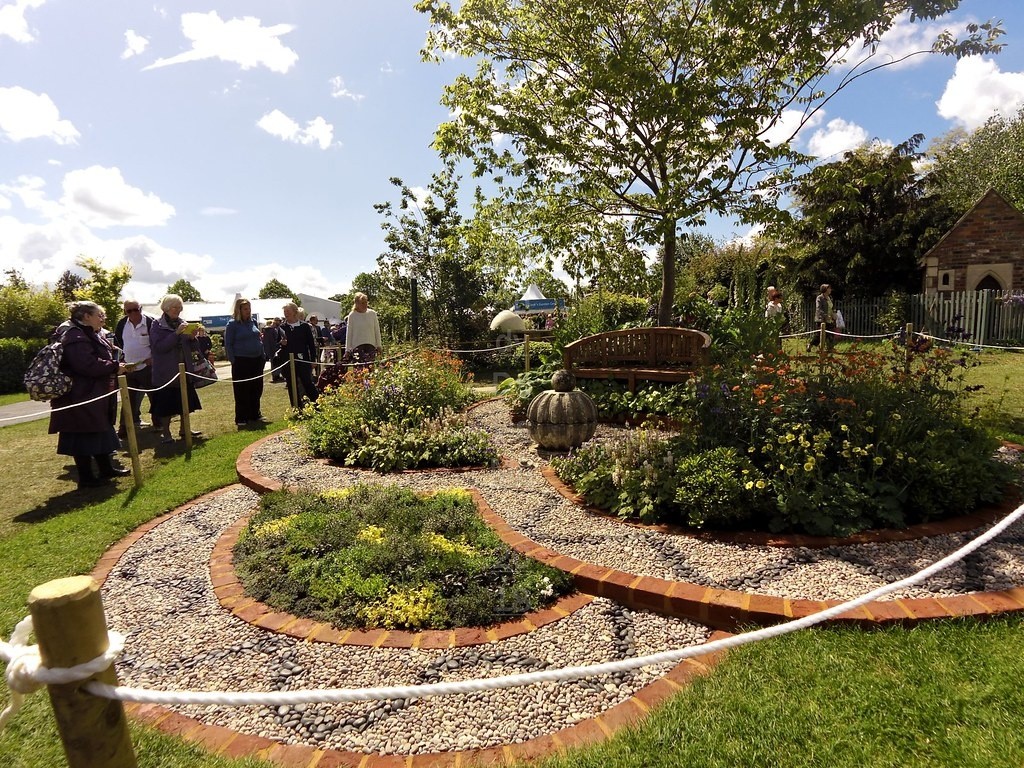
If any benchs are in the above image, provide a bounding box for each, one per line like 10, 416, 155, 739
564, 326, 711, 392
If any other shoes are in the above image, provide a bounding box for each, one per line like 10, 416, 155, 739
84, 478, 106, 486
139, 421, 150, 426
99, 468, 130, 477
259, 416, 266, 420
179, 430, 203, 436
163, 435, 175, 443
236, 421, 246, 425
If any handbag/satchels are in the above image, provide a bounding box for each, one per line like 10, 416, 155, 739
273, 349, 282, 376
192, 348, 218, 389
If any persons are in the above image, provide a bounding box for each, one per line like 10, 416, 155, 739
320, 319, 335, 344
344, 291, 384, 373
276, 302, 322, 420
765, 287, 784, 350
258, 314, 347, 361
47, 301, 130, 477
805, 284, 841, 353
150, 295, 202, 442
522, 308, 570, 330
114, 299, 164, 434
297, 307, 318, 358
224, 299, 273, 426
194, 326, 212, 362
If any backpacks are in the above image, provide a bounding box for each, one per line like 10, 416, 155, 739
25, 326, 83, 402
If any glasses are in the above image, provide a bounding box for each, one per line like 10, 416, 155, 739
94, 314, 102, 318
126, 308, 140, 314
172, 306, 183, 310
